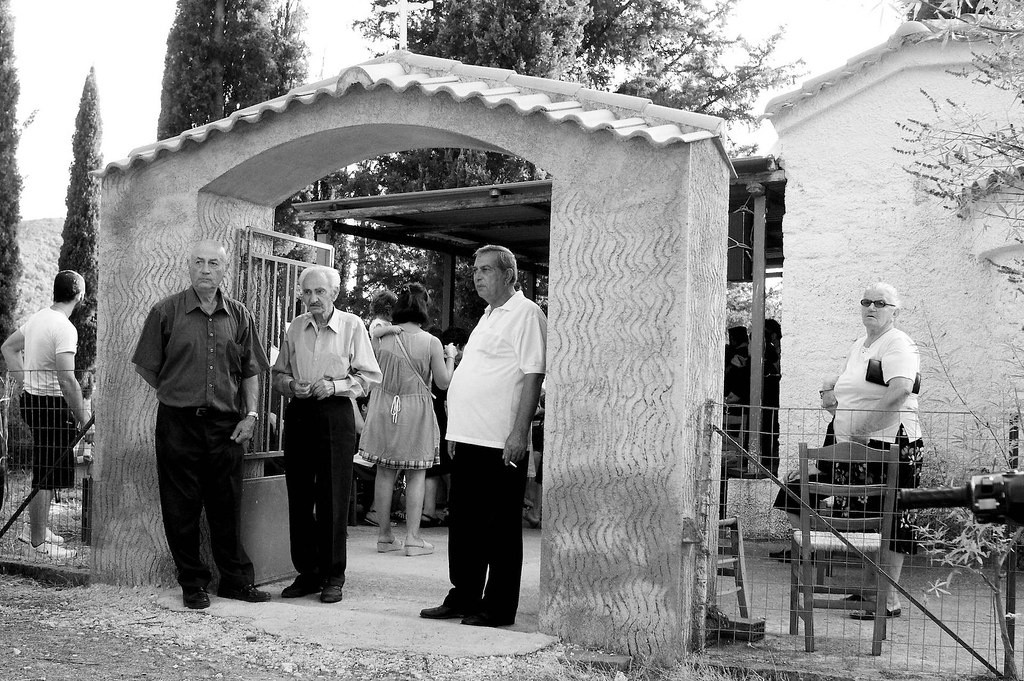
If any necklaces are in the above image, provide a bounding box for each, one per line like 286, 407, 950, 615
54, 305, 69, 318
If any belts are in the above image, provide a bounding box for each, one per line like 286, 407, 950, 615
176, 405, 246, 420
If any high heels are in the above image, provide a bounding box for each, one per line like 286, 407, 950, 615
375, 536, 402, 551
404, 538, 435, 556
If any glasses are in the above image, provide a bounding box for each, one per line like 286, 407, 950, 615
861, 298, 896, 309
819, 388, 834, 399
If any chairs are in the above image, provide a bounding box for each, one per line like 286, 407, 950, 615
716, 509, 753, 624
788, 440, 902, 657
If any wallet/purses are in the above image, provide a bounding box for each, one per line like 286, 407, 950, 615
866, 359, 921, 394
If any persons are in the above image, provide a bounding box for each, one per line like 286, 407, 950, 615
821, 283, 924, 620
420, 245, 546, 627
353, 282, 470, 528
131, 240, 271, 610
270, 265, 383, 603
1, 270, 95, 567
725, 319, 781, 461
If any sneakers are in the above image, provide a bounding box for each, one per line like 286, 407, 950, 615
18, 524, 65, 546
390, 510, 407, 522
27, 541, 77, 560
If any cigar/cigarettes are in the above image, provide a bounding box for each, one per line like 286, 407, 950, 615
509, 461, 517, 468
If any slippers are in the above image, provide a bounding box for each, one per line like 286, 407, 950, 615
844, 594, 876, 602
850, 606, 902, 620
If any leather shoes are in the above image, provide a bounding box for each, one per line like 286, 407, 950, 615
319, 585, 342, 602
460, 616, 490, 627
420, 604, 463, 619
182, 588, 210, 609
216, 586, 270, 602
280, 581, 322, 599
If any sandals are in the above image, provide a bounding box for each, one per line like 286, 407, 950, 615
365, 511, 398, 527
420, 513, 446, 529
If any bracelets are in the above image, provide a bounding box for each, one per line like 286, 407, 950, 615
447, 355, 455, 359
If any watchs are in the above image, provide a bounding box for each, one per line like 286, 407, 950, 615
246, 412, 260, 421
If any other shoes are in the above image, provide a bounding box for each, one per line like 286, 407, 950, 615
769, 548, 819, 564
